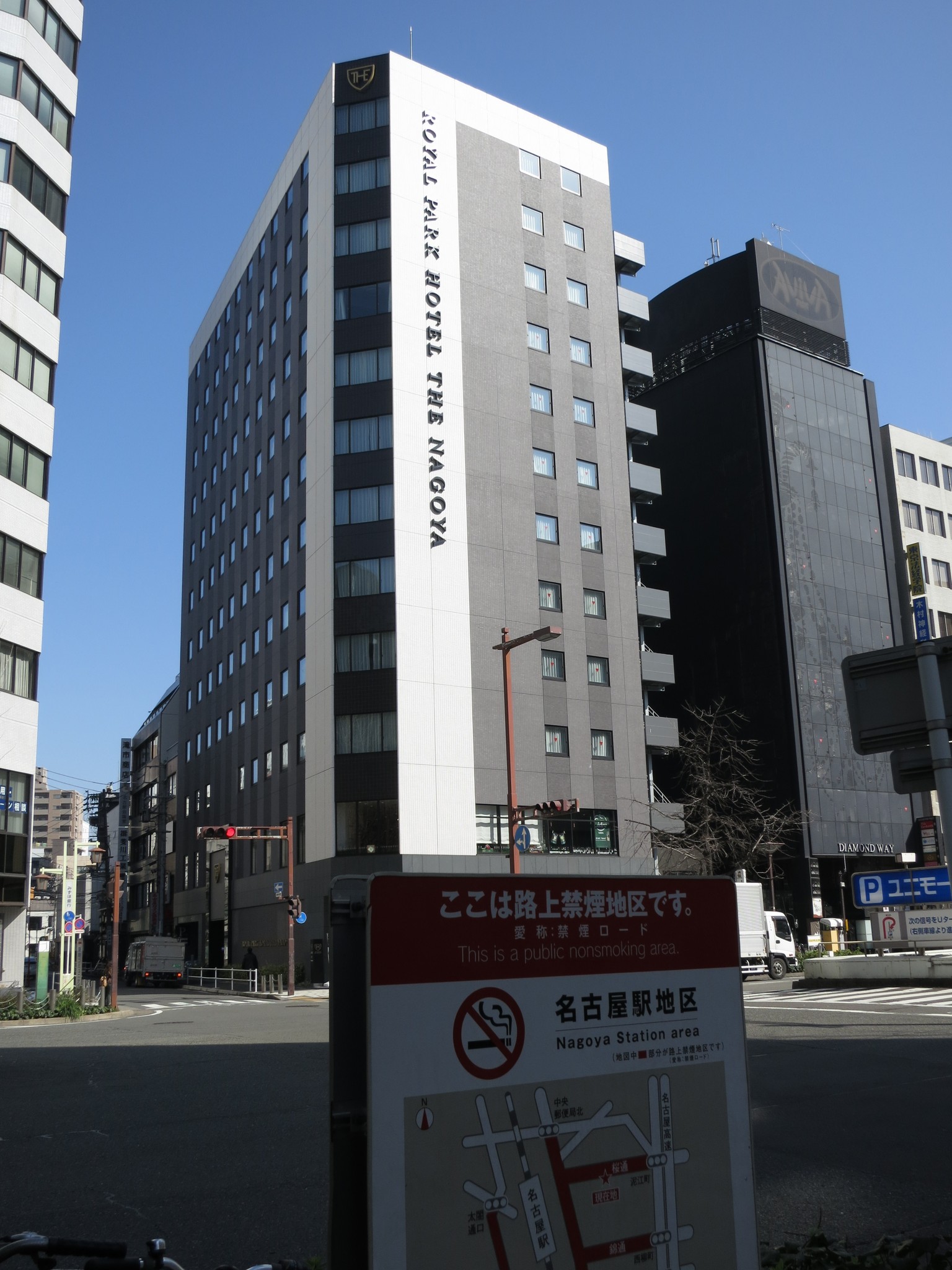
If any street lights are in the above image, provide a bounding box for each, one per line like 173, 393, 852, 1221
33, 840, 106, 998
491, 625, 562, 873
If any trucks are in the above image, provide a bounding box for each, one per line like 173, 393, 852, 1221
735, 881, 798, 982
123, 936, 187, 988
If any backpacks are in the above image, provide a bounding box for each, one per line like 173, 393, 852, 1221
100, 976, 107, 987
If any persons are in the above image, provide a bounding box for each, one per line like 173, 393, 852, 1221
103, 961, 113, 1006
243, 948, 259, 987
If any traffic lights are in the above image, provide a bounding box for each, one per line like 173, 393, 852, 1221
287, 898, 301, 918
201, 826, 238, 840
535, 799, 572, 814
107, 880, 124, 900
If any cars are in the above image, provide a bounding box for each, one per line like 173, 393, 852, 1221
24, 956, 37, 978
